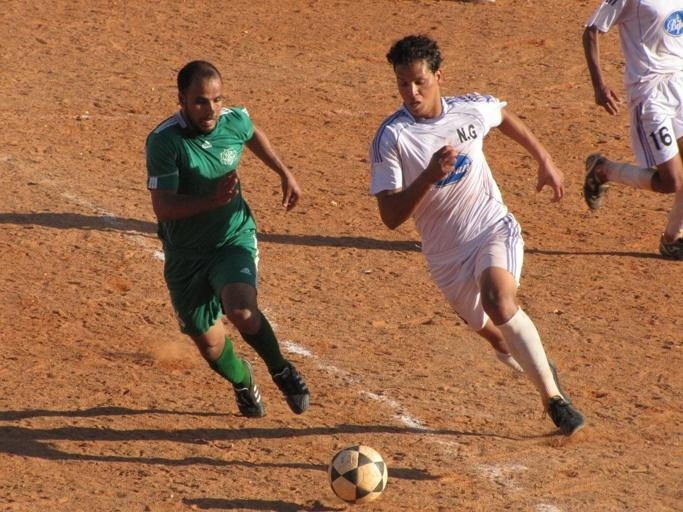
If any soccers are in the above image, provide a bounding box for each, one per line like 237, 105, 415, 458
327, 445, 389, 506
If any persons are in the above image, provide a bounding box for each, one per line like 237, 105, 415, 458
145, 60, 310, 418
369, 35, 588, 439
583, 0, 683, 260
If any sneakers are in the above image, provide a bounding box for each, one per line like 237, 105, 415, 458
272, 365, 309, 415
584, 154, 607, 211
659, 234, 683, 260
233, 359, 264, 417
549, 362, 586, 436
